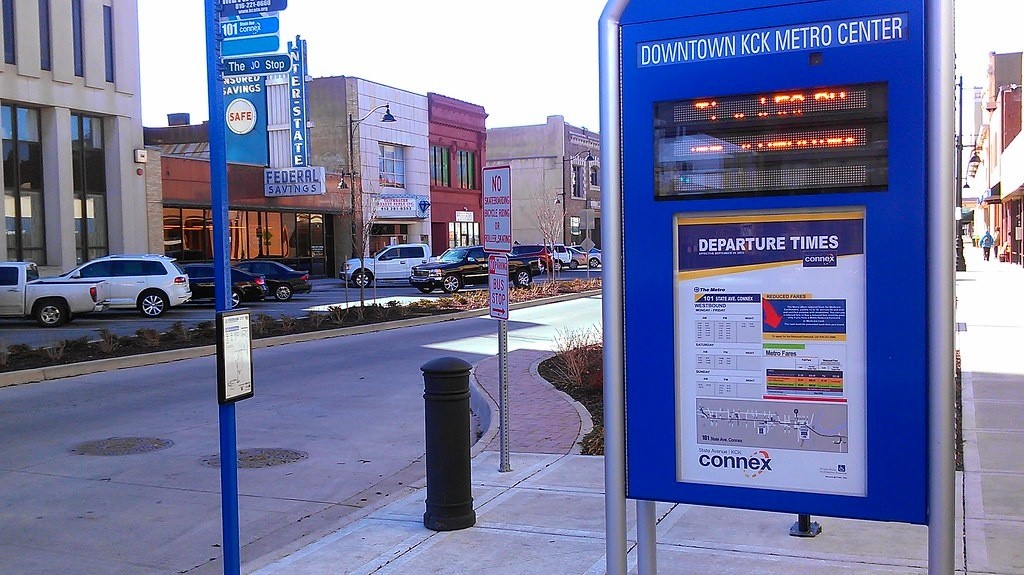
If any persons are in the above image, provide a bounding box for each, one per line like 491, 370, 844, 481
993, 227, 1000, 258
981, 230, 994, 261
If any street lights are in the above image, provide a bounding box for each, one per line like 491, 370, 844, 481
955, 73, 981, 272
336, 102, 397, 259
554, 148, 595, 246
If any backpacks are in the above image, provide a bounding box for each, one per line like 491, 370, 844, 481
984, 237, 990, 245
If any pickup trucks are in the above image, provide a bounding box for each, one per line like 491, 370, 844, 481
0, 262, 112, 328
339, 244, 437, 288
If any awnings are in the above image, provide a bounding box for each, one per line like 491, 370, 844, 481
977, 183, 1001, 204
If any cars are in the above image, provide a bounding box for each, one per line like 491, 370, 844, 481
565, 247, 587, 270
230, 261, 313, 302
569, 245, 601, 268
409, 245, 542, 294
510, 243, 553, 276
181, 264, 267, 307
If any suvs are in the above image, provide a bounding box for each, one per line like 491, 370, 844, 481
534, 244, 572, 272
56, 253, 193, 317
